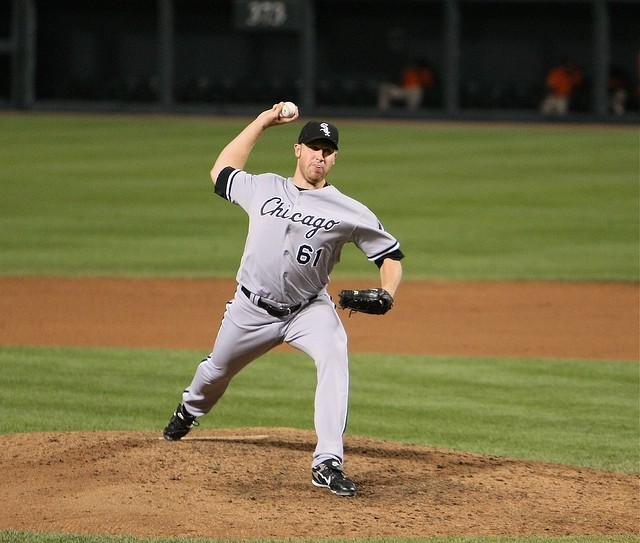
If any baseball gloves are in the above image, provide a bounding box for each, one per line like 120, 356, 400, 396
337, 287, 393, 314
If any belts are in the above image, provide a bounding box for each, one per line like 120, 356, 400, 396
239, 284, 318, 317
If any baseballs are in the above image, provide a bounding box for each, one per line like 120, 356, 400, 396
281, 101, 297, 118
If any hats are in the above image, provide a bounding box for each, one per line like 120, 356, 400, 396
298, 121, 339, 151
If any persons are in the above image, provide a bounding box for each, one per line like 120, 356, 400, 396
376, 54, 435, 113
539, 57, 584, 116
607, 65, 628, 117
162, 101, 405, 497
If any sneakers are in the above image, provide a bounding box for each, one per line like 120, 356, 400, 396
311, 458, 356, 494
163, 403, 200, 441
